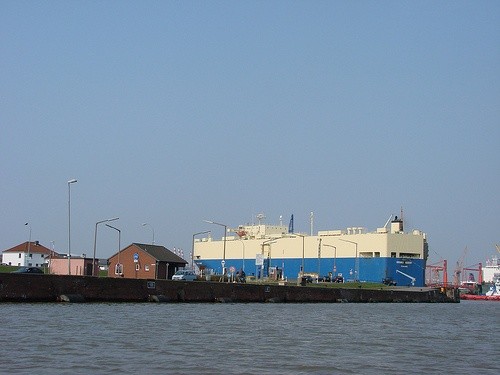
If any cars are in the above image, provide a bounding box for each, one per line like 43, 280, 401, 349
331, 276, 344, 283
172, 269, 196, 281
11, 267, 44, 274
382, 277, 397, 286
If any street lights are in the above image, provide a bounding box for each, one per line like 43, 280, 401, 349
24, 222, 32, 268
202, 220, 227, 275
92, 217, 120, 278
323, 244, 337, 278
260, 236, 296, 283
105, 223, 120, 278
142, 223, 154, 245
339, 239, 358, 281
296, 233, 304, 276
67, 179, 78, 275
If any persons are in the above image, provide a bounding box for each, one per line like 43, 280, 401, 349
491, 283, 498, 296
475, 284, 482, 295
235, 268, 246, 283
